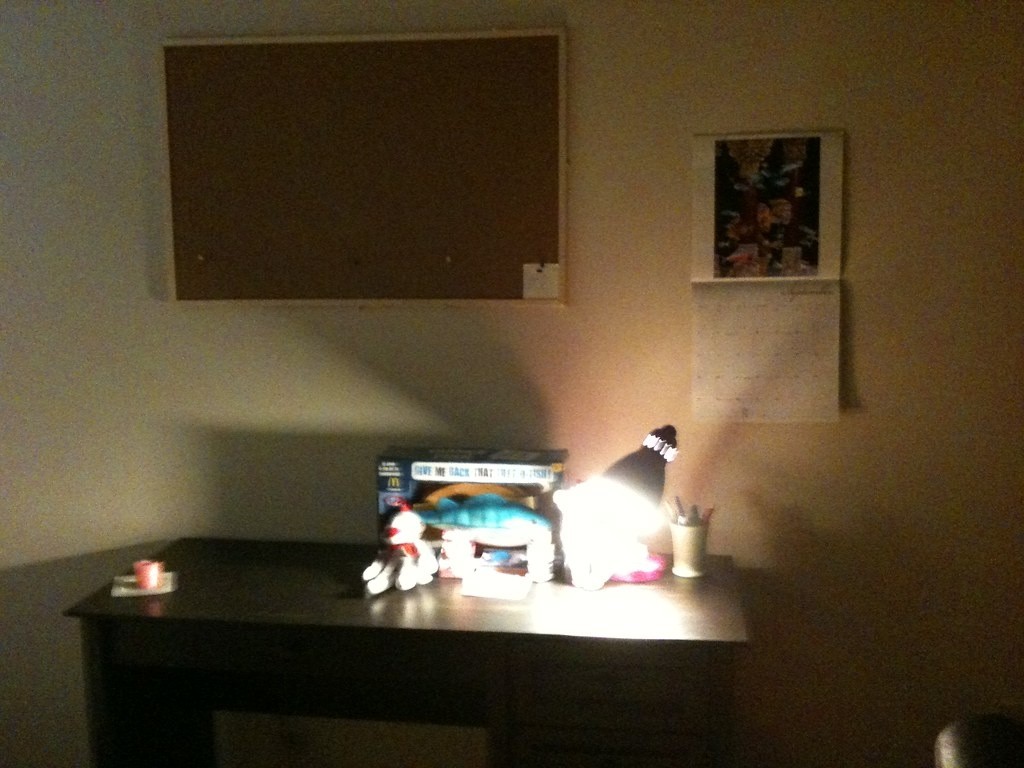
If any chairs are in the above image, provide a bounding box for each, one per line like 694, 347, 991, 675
934, 714, 1024, 768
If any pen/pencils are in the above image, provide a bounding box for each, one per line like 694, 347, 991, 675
662, 495, 717, 526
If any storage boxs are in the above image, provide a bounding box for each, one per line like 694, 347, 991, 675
376, 444, 567, 583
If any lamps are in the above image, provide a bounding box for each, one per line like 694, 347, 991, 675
553, 424, 679, 591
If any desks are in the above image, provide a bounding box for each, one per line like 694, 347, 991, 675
64, 538, 749, 768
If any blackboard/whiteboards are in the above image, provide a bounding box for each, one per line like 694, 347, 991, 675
157, 23, 568, 309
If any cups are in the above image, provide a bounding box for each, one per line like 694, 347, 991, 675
133, 560, 158, 589
668, 519, 710, 579
